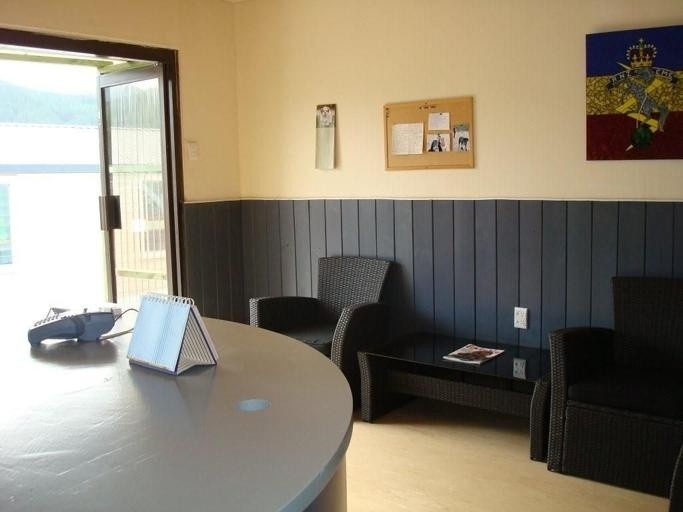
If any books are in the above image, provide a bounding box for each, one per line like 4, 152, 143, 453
442, 343, 505, 366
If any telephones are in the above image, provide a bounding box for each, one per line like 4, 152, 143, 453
28, 304, 121, 347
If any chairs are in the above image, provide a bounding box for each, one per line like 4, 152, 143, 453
248, 253, 395, 408
543, 272, 681, 500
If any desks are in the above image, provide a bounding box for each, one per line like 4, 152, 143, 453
0, 305, 354, 512
